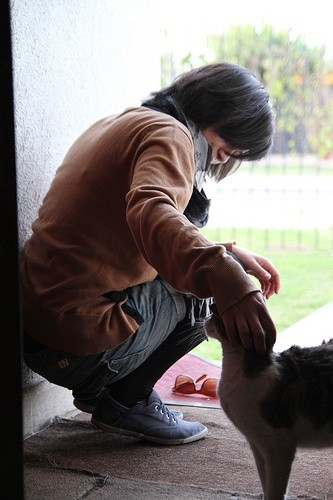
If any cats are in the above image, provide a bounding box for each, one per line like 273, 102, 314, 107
173, 297, 333, 500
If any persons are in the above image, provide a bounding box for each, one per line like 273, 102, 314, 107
21, 62, 281, 445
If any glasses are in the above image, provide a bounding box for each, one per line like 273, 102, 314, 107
172, 374, 219, 399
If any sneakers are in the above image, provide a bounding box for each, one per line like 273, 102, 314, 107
74, 389, 99, 414
91, 389, 205, 444
